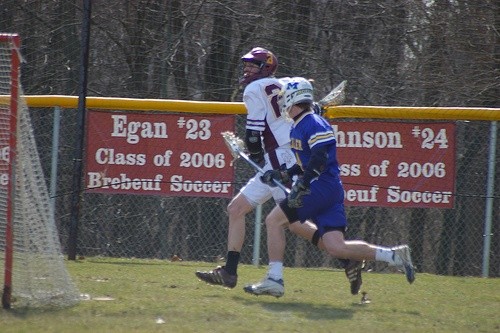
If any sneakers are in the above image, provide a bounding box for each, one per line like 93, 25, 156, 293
196, 266, 237, 290
345, 259, 365, 295
244, 272, 285, 298
391, 244, 415, 284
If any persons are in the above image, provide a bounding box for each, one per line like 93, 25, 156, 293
195, 46, 362, 289
242, 76, 416, 298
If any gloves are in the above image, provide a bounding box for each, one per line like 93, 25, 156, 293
249, 149, 265, 168
260, 164, 303, 187
287, 179, 312, 208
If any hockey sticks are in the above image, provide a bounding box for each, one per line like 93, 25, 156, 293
231, 80, 348, 166
220, 131, 291, 195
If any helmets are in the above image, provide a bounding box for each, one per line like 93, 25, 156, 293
279, 76, 313, 109
239, 47, 278, 89
